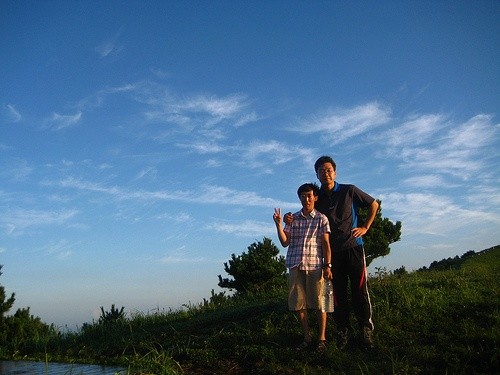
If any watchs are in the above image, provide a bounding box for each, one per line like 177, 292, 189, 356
323, 263, 332, 268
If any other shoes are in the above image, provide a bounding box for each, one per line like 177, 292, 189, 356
295, 340, 313, 351
317, 339, 327, 353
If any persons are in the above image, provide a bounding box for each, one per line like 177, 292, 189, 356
283, 156, 379, 350
272, 183, 333, 352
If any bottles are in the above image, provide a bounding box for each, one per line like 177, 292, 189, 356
321, 279, 334, 313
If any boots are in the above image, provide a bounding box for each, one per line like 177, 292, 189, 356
361, 327, 376, 350
337, 328, 355, 350
